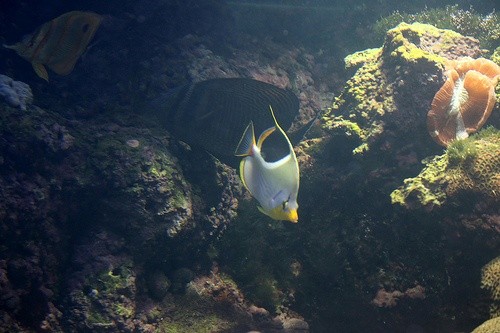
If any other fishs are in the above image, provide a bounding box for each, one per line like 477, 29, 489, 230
3, 11, 105, 89
234, 104, 300, 223
150, 78, 319, 175
